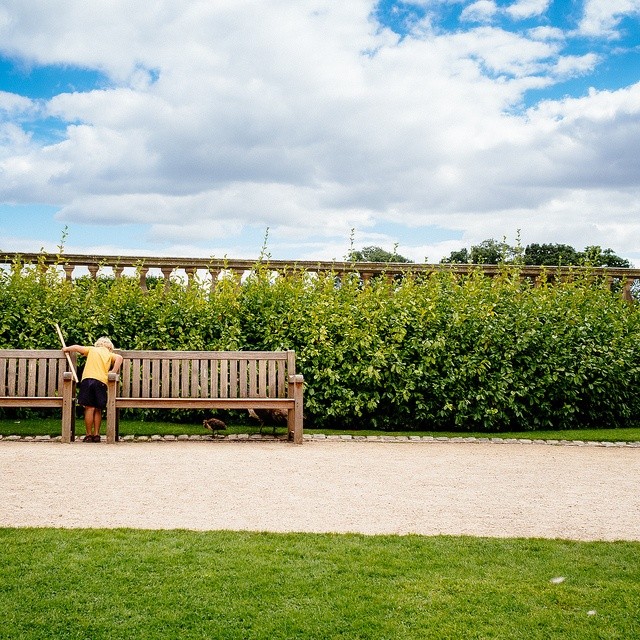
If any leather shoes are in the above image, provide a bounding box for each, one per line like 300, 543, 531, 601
83, 435, 92, 442
92, 435, 101, 443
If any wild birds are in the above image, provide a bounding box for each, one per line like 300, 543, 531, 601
201, 419, 228, 435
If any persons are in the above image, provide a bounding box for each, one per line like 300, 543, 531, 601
61, 336, 123, 442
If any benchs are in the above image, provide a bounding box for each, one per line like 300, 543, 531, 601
0, 349, 78, 444
106, 347, 304, 445
